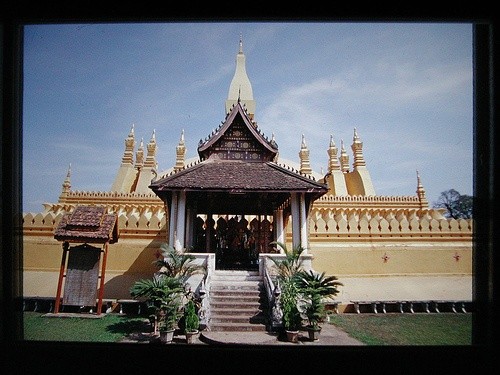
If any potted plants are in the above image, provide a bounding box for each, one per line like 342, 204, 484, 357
126, 241, 209, 346
266, 240, 345, 341
